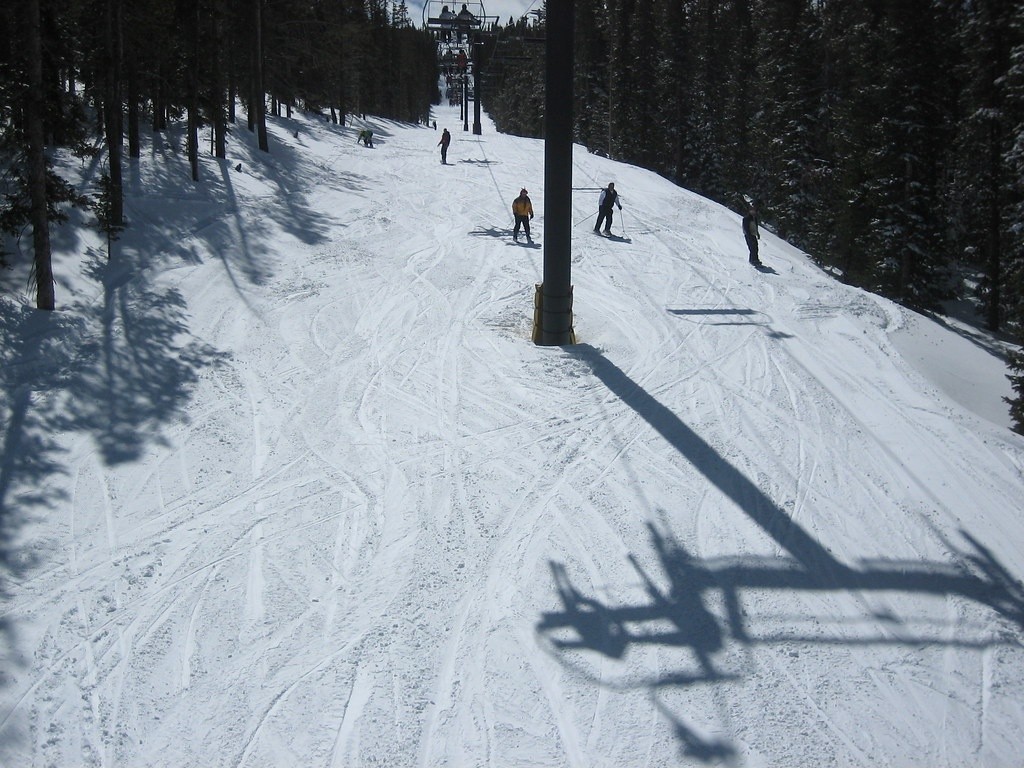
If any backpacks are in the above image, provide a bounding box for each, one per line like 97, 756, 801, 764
366, 130, 373, 138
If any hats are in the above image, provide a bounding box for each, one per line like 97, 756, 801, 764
520, 186, 528, 195
608, 183, 614, 188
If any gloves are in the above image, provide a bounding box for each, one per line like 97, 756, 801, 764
618, 204, 622, 210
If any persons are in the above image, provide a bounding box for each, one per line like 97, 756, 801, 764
512, 189, 534, 244
742, 205, 766, 269
594, 183, 623, 236
437, 5, 479, 86
437, 128, 451, 163
357, 130, 373, 147
433, 120, 436, 130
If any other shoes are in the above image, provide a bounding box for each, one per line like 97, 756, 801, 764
440, 160, 447, 164
603, 230, 612, 236
365, 144, 373, 148
593, 229, 601, 236
527, 237, 534, 245
513, 238, 519, 243
